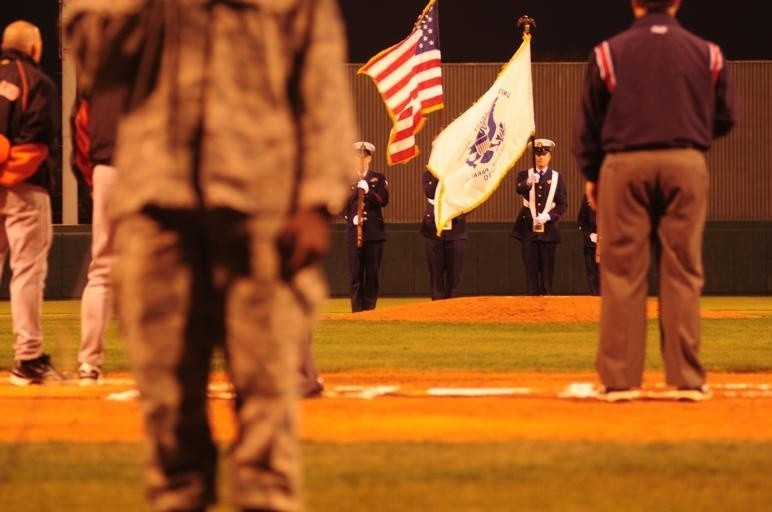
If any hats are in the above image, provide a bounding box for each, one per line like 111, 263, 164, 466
352, 141, 376, 156
527, 138, 556, 153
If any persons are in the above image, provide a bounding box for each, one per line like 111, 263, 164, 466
342, 140, 390, 314
420, 171, 468, 301
572, 0, 741, 400
300, 333, 321, 397
577, 194, 600, 296
511, 139, 568, 295
64, 0, 361, 512
69, 96, 117, 386
0, 19, 70, 386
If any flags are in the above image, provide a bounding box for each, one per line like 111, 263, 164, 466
356, 0, 445, 167
426, 34, 536, 238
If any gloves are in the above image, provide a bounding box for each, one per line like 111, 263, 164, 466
353, 215, 363, 225
590, 232, 598, 243
357, 179, 370, 194
533, 212, 552, 226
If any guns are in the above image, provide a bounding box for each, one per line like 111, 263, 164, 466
358, 143, 366, 246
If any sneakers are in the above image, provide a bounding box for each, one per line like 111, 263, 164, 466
10, 351, 69, 388
603, 387, 637, 402
673, 384, 714, 401
76, 358, 103, 387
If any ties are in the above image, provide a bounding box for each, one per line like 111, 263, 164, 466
539, 170, 544, 176
